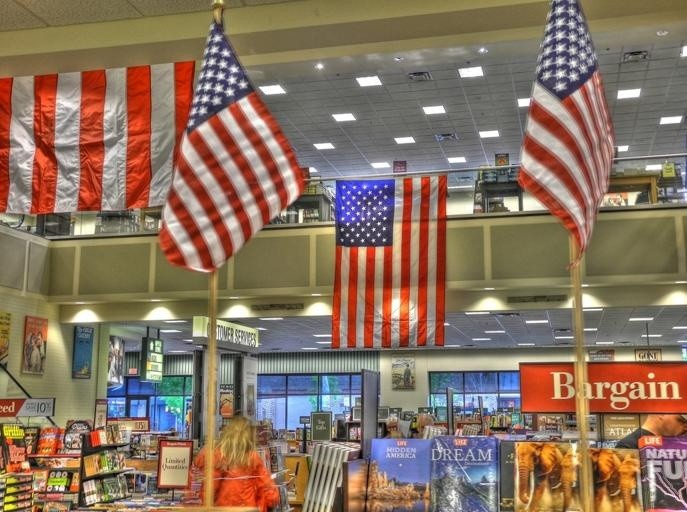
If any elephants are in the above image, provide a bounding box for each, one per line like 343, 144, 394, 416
589, 448, 644, 512
516, 442, 580, 512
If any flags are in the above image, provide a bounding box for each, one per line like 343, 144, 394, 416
0, 59, 195, 221
328, 177, 449, 350
152, 1, 307, 280
514, 1, 617, 276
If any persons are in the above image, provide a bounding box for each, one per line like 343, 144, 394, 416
25, 332, 42, 373
192, 416, 282, 512
36, 330, 46, 372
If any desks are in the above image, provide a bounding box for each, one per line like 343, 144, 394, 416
479, 179, 527, 212
139, 206, 162, 231
285, 193, 333, 224
606, 170, 664, 205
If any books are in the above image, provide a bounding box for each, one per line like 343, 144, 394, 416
0, 420, 201, 512
254, 403, 686, 512
